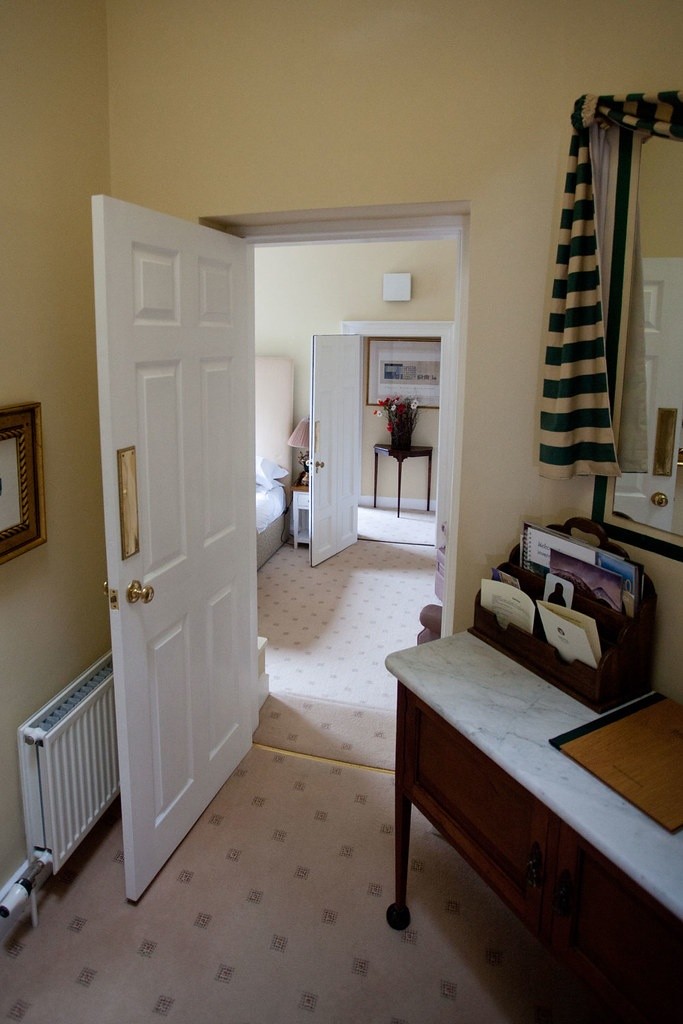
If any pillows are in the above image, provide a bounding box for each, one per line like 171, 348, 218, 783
256, 456, 289, 491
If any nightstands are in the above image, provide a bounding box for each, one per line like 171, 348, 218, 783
289, 486, 310, 550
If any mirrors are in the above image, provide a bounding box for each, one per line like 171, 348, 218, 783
592, 125, 683, 565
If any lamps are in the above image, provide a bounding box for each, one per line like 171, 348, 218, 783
288, 417, 310, 486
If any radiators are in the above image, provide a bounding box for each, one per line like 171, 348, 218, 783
18, 649, 121, 877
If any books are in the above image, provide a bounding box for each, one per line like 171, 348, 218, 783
521, 521, 638, 617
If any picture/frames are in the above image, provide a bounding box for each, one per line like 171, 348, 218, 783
0, 400, 47, 567
366, 337, 439, 409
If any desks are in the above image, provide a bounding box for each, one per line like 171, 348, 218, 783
373, 443, 433, 519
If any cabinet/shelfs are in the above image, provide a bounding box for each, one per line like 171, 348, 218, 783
385, 629, 683, 1024
469, 516, 656, 715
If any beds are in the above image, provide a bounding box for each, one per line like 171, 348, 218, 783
256, 354, 294, 572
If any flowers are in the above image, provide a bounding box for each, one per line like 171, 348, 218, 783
372, 393, 423, 438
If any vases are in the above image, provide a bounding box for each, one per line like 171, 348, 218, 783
391, 434, 412, 452
